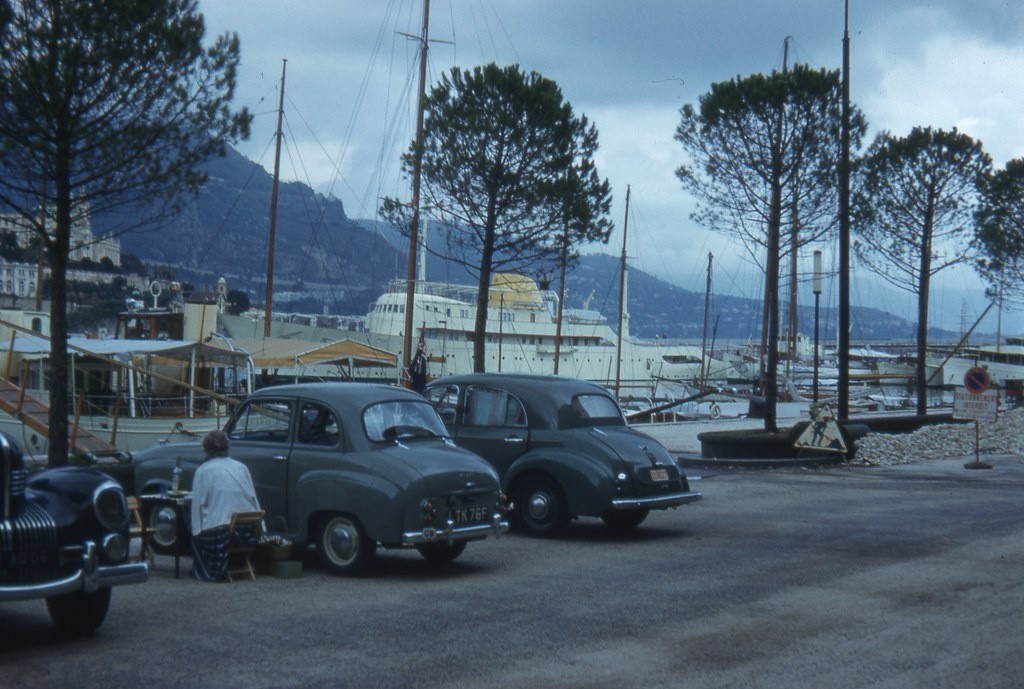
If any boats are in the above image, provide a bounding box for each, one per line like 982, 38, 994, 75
0, 0, 1024, 465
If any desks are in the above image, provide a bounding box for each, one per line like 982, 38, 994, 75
139, 490, 193, 579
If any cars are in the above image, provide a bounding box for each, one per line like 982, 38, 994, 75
0, 428, 154, 639
130, 381, 515, 574
358, 372, 704, 536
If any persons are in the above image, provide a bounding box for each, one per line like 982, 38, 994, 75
812, 416, 829, 448
191, 429, 268, 580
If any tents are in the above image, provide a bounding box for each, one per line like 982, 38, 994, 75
0, 334, 251, 416
134, 336, 398, 386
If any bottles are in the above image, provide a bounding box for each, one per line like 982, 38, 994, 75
172, 455, 183, 491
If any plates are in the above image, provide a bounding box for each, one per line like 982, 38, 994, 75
168, 491, 188, 497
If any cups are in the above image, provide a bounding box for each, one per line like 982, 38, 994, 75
159, 479, 168, 499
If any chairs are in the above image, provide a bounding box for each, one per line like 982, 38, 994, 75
190, 509, 266, 584
127, 496, 157, 569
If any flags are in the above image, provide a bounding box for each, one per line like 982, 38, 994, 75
411, 325, 427, 393
